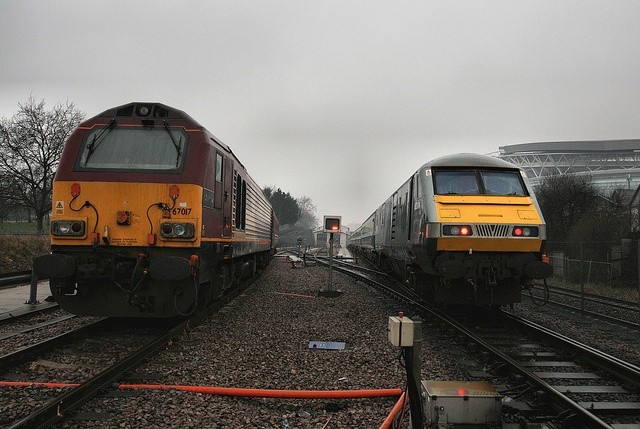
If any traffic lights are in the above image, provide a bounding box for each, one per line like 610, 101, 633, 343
322, 215, 343, 232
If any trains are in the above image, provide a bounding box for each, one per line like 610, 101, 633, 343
344, 152, 559, 317
48, 100, 277, 332
